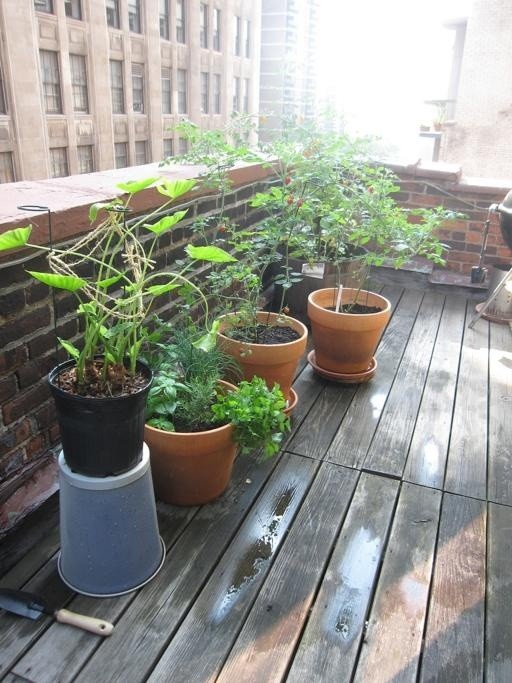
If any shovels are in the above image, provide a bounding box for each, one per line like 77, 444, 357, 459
0, 589, 114, 639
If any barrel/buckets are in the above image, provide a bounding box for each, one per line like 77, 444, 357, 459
272, 239, 370, 326
58, 442, 167, 599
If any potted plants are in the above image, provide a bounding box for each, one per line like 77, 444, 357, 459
141, 318, 294, 506
161, 113, 335, 409
0, 174, 239, 478
299, 122, 467, 381
252, 112, 393, 326
